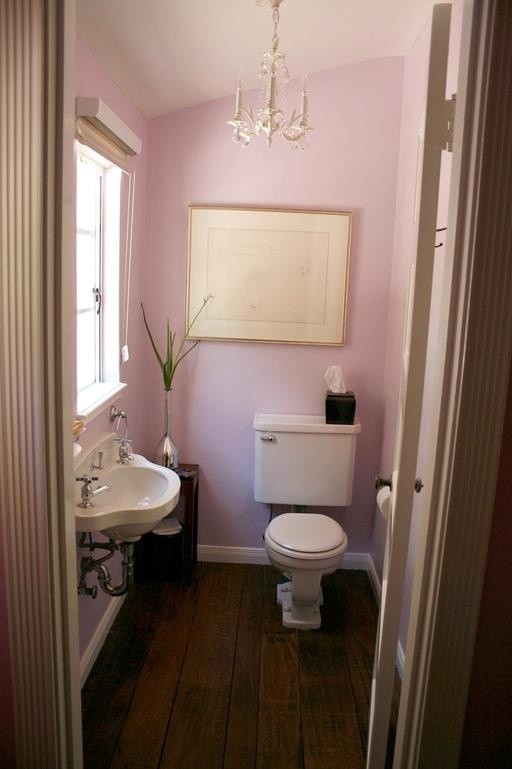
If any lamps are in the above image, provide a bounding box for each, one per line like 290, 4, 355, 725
228, 0, 315, 153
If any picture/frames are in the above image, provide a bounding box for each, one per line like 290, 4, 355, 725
184, 203, 353, 347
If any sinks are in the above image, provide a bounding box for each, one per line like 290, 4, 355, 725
75, 454, 182, 542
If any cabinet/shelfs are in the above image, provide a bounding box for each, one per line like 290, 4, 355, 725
130, 467, 200, 578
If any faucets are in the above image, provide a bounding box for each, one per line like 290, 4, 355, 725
76, 476, 111, 509
115, 439, 134, 465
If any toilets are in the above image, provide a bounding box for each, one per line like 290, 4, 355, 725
251, 411, 361, 633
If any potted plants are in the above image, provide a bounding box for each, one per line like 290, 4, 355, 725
137, 291, 218, 466
